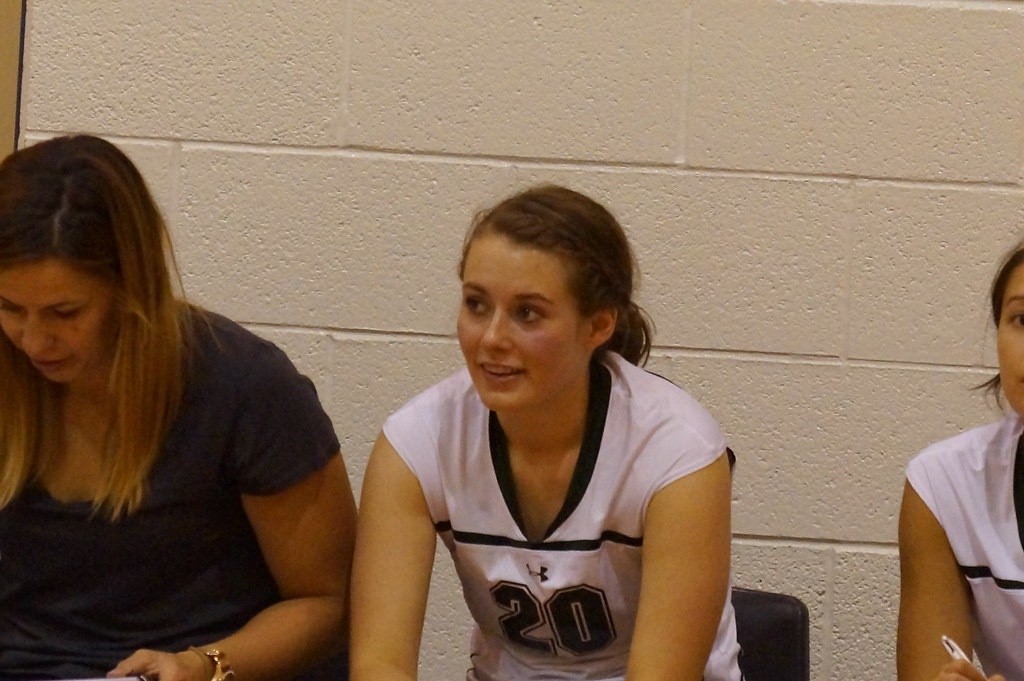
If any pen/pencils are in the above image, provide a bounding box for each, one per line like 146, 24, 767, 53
940, 634, 973, 667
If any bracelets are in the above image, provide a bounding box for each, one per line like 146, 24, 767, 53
187, 645, 209, 681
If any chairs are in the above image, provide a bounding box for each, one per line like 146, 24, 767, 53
731, 584, 811, 681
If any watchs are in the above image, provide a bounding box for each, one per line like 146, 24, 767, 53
195, 645, 238, 681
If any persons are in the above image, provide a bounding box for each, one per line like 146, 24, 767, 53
0, 136, 355, 681
896, 240, 1024, 681
348, 187, 747, 681
